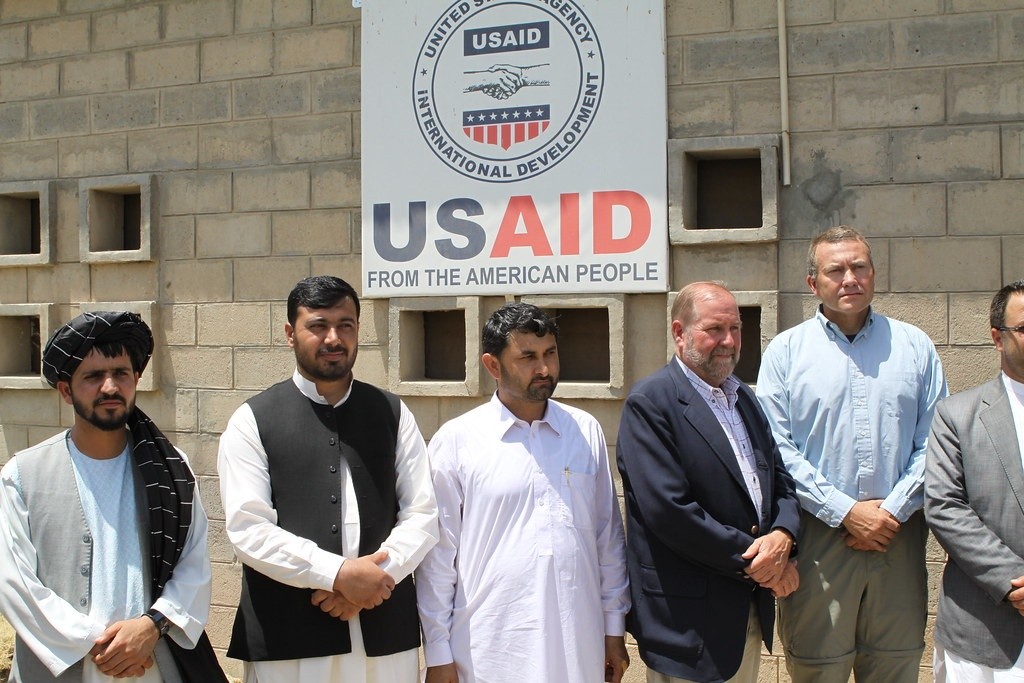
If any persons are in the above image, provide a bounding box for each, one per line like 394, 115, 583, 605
614, 281, 806, 683
414, 302, 633, 683
755, 223, 951, 683
216, 275, 441, 683
0, 311, 230, 683
922, 284, 1024, 683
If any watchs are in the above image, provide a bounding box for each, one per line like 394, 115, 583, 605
140, 610, 170, 640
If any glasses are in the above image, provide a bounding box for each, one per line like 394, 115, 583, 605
999, 325, 1024, 338
